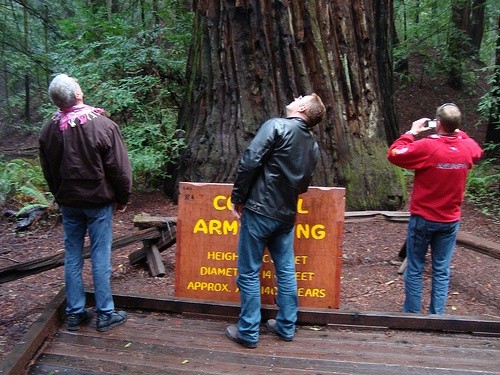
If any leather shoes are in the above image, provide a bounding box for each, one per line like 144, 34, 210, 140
96, 311, 127, 331
226, 326, 258, 348
267, 318, 295, 340
67, 307, 96, 331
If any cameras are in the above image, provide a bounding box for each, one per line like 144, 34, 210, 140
428, 118, 437, 127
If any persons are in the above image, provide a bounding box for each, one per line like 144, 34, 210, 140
37, 73, 134, 332
225, 93, 325, 349
387, 102, 483, 315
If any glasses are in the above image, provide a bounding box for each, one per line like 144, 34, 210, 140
298, 95, 307, 108
